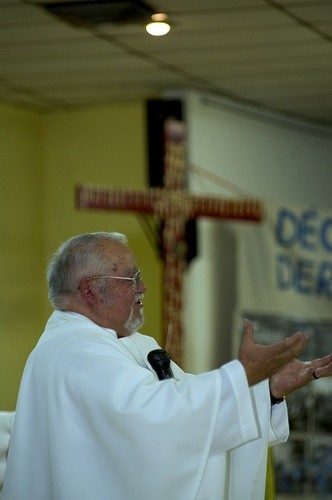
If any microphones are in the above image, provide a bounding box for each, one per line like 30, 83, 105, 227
147, 349, 173, 381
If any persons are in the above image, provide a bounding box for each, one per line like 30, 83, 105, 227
0, 232, 332, 500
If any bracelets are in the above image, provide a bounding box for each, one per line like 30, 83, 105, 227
270, 389, 284, 404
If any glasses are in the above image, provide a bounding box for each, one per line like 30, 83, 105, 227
76, 268, 141, 292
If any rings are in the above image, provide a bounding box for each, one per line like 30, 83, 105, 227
313, 371, 318, 379
